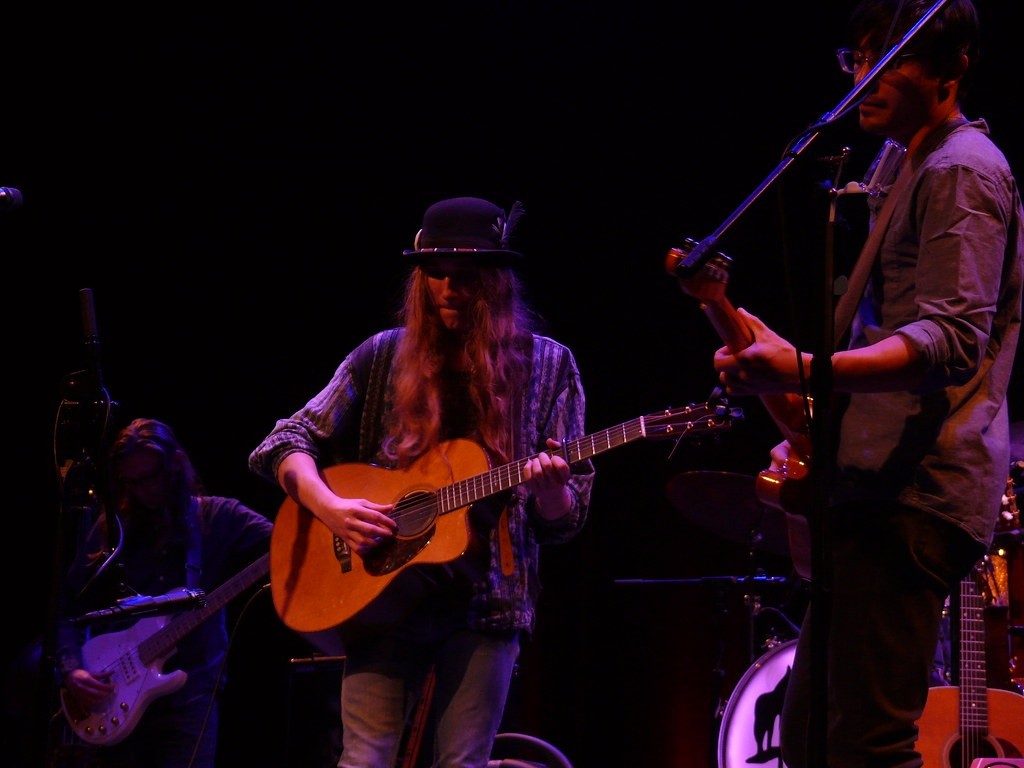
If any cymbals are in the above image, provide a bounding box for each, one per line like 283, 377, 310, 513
666, 468, 786, 546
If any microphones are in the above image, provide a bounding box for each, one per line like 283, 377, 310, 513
0, 187, 23, 208
74, 590, 207, 624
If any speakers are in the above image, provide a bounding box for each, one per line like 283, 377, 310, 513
289, 655, 347, 768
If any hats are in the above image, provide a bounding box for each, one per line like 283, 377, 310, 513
403, 197, 526, 259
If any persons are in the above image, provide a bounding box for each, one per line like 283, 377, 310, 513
713, 0, 1024, 768
50, 417, 273, 768
248, 197, 596, 768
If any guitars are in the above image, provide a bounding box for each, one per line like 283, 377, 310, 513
665, 235, 825, 585
60, 549, 270, 746
266, 396, 750, 660
913, 555, 1024, 768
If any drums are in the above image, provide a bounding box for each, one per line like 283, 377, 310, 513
988, 454, 1024, 541
709, 631, 802, 768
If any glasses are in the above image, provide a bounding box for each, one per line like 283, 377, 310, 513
837, 47, 921, 73
